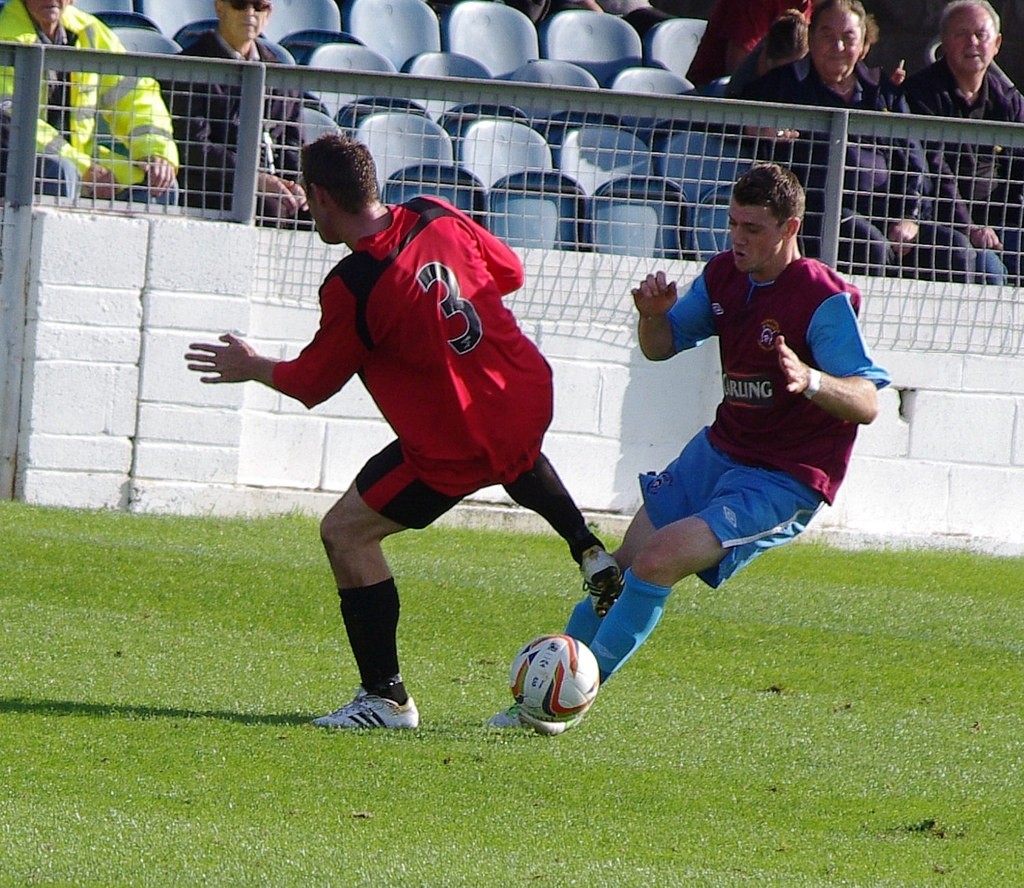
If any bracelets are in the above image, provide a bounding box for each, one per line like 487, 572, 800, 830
804, 367, 822, 401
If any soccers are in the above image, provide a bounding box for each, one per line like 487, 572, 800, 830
511, 633, 600, 723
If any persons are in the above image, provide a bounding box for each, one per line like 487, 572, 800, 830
184, 139, 626, 728
0, 0, 180, 207
502, 0, 1024, 287
488, 164, 892, 730
163, 0, 316, 231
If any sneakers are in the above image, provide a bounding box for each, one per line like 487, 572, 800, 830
519, 705, 582, 736
579, 546, 624, 617
310, 690, 418, 727
487, 703, 522, 728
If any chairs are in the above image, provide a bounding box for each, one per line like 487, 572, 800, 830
35, 0, 761, 260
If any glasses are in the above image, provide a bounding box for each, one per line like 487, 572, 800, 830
228, 0, 272, 11
